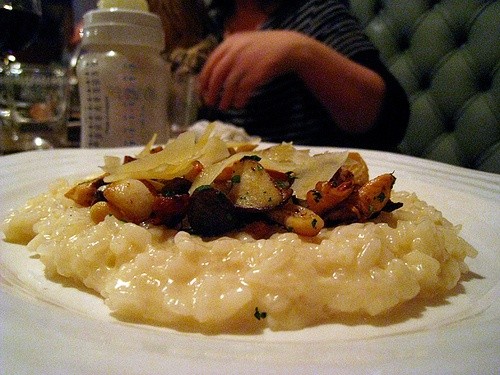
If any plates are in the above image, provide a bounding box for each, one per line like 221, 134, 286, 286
0, 143, 500, 375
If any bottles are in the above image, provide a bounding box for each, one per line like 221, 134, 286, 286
76, 0, 170, 149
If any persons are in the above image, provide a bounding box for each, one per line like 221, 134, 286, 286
189, 0, 410, 155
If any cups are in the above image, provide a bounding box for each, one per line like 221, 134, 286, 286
0, 63, 71, 151
